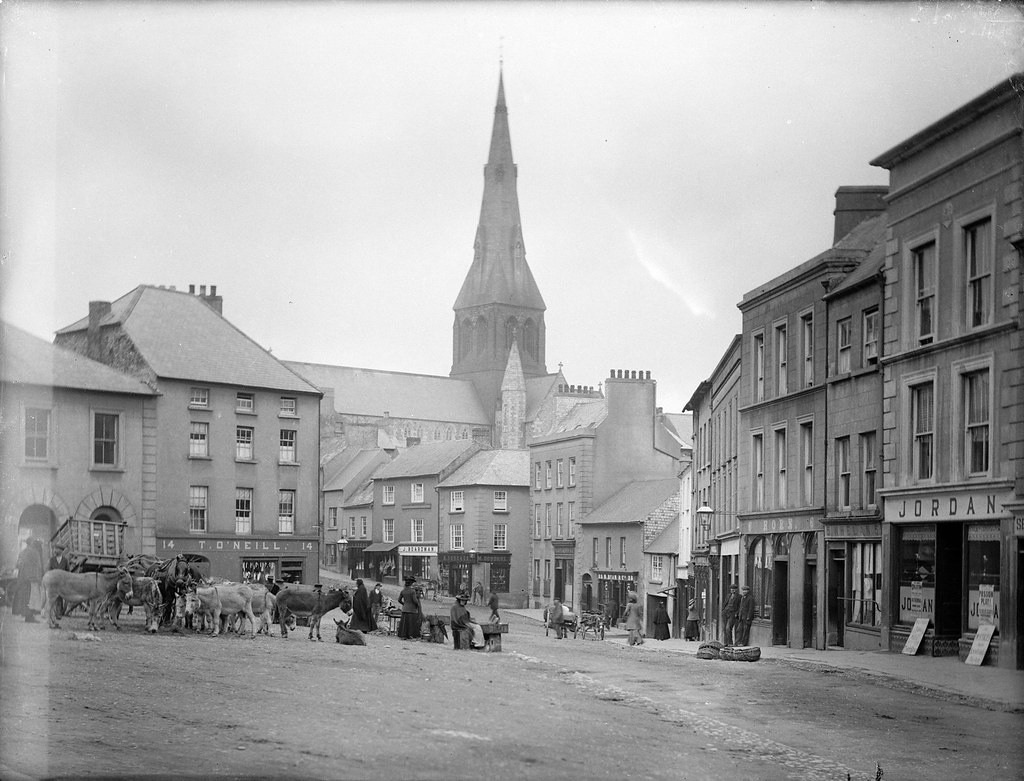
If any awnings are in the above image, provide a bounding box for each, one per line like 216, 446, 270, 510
362, 542, 399, 551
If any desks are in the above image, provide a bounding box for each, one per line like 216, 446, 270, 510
386, 612, 401, 636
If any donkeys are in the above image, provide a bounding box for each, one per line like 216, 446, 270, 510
108, 555, 297, 638
39, 567, 133, 630
276, 585, 352, 642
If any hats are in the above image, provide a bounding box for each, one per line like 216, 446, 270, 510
456, 592, 469, 601
404, 576, 416, 582
729, 584, 737, 588
742, 586, 750, 590
689, 599, 694, 604
658, 602, 663, 607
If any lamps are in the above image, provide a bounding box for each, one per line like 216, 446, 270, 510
694, 501, 745, 531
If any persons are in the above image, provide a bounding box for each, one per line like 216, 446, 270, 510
652, 602, 671, 641
487, 591, 500, 621
451, 593, 485, 649
266, 576, 288, 623
473, 582, 483, 606
397, 576, 422, 640
685, 599, 700, 641
623, 591, 643, 645
350, 579, 378, 635
13, 537, 45, 623
46, 543, 70, 619
552, 597, 563, 639
608, 598, 618, 626
721, 585, 755, 646
604, 604, 610, 631
369, 583, 383, 622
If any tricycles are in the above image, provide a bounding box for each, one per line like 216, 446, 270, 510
580, 609, 608, 641
542, 606, 580, 640
